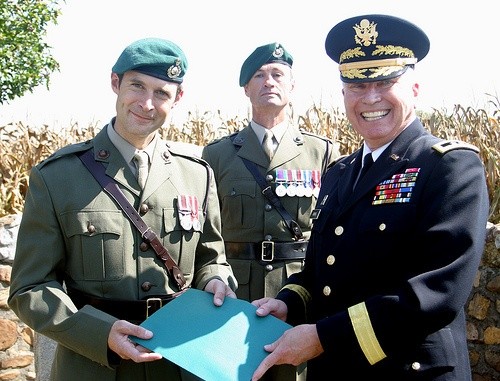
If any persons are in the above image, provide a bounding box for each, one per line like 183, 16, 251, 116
201, 42, 340, 381
8, 37, 237, 381
252, 13, 489, 381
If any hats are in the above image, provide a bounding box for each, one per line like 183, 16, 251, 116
239, 43, 293, 87
324, 14, 430, 82
112, 38, 189, 83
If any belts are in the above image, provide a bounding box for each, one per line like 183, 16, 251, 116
224, 241, 307, 261
67, 286, 191, 322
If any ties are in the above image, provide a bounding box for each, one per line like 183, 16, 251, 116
353, 153, 374, 195
